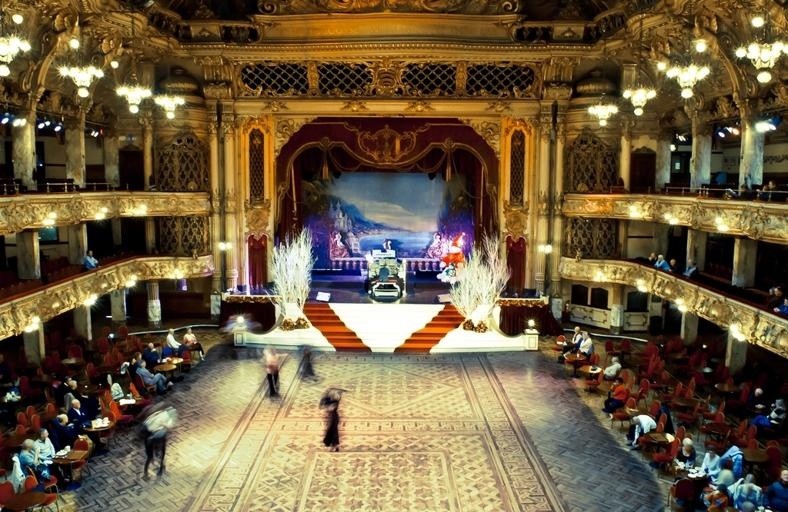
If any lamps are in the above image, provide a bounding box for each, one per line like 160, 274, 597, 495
35, 114, 64, 133
154, 37, 185, 118
10, 115, 21, 127
666, 5, 711, 98
1, 113, 9, 126
622, 12, 657, 116
769, 115, 781, 131
85, 127, 95, 138
588, 32, 618, 126
677, 131, 692, 142
57, 60, 104, 99
736, 15, 788, 85
718, 127, 731, 139
116, 13, 152, 114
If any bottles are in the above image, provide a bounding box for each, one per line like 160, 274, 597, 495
685, 459, 689, 470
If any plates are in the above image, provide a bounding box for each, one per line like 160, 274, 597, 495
54, 450, 69, 458
96, 417, 109, 428
686, 466, 706, 478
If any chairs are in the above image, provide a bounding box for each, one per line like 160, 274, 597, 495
1, 327, 201, 511
551, 335, 781, 512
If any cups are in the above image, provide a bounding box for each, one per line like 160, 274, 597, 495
577, 352, 580, 357
127, 394, 131, 399
7, 391, 16, 400
65, 445, 71, 452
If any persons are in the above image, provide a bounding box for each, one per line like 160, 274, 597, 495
602, 378, 627, 421
727, 181, 786, 202
627, 415, 657, 450
591, 357, 621, 381
648, 253, 700, 281
747, 388, 787, 432
19, 327, 204, 493
559, 327, 593, 377
674, 438, 788, 512
251, 344, 348, 451
769, 286, 786, 317
84, 250, 98, 269
